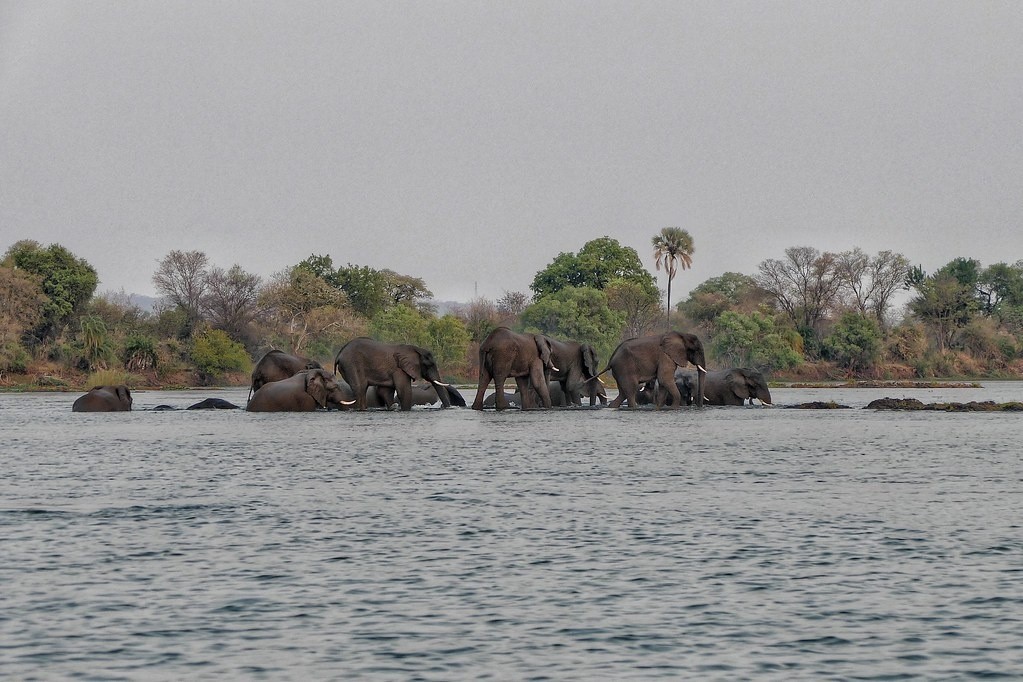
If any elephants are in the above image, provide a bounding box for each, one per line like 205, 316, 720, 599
243, 326, 772, 414
72, 385, 133, 412
